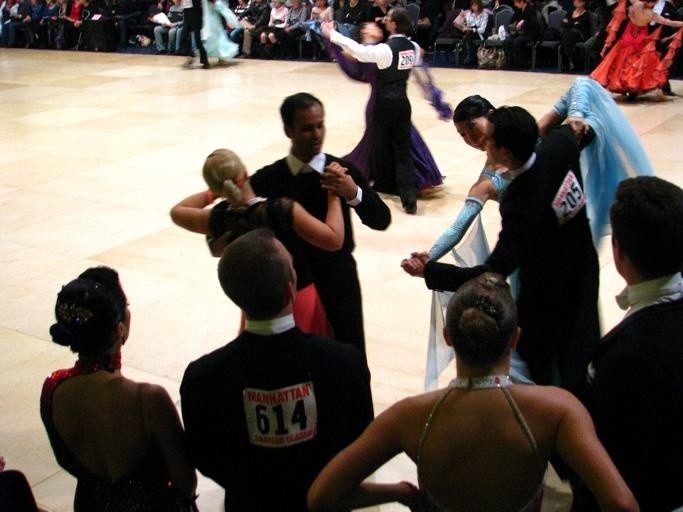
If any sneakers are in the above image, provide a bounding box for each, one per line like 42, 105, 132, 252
406, 197, 417, 213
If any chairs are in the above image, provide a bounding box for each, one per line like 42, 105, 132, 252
531, 10, 598, 73
432, 8, 464, 66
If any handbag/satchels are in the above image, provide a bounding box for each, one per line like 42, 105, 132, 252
477, 47, 497, 68
252, 42, 271, 58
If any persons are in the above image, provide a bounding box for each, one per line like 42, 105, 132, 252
452, 0, 489, 66
400, 78, 653, 389
560, 0, 593, 75
39, 265, 198, 510
581, 175, 683, 512
322, 6, 452, 216
310, 273, 640, 512
503, 0, 538, 69
0, 0, 448, 69
589, 0, 683, 101
180, 228, 373, 511
170, 94, 392, 425
